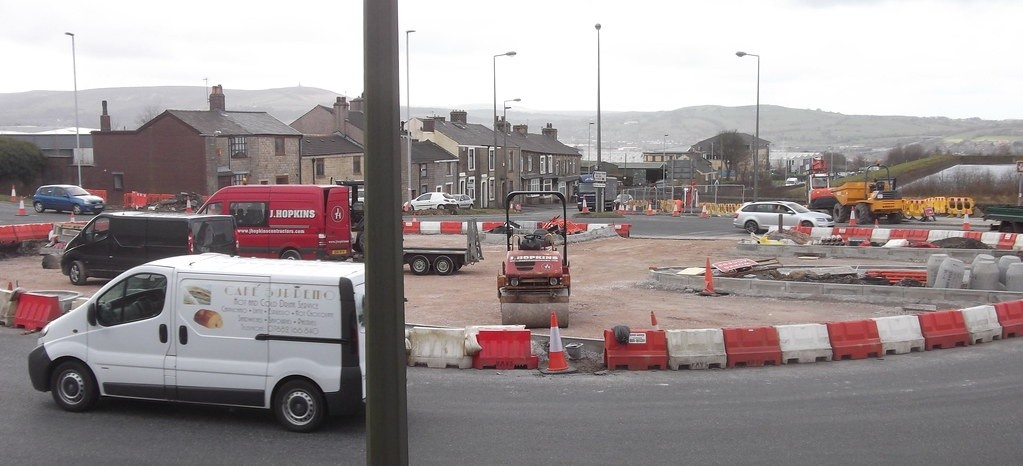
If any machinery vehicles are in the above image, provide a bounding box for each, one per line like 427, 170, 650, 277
496, 189, 572, 328
806, 155, 908, 224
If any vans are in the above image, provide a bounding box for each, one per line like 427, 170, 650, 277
30, 252, 365, 432
58, 184, 364, 287
784, 177, 799, 189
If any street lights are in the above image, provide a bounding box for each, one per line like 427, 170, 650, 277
406, 30, 417, 216
595, 23, 601, 208
493, 50, 518, 208
65, 32, 82, 187
503, 98, 522, 209
663, 135, 669, 201
588, 122, 595, 178
734, 51, 760, 201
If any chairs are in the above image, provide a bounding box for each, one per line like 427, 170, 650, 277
231, 207, 255, 228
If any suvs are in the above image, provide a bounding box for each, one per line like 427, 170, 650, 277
33, 184, 106, 216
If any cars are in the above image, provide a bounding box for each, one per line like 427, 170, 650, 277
733, 200, 836, 235
403, 192, 475, 214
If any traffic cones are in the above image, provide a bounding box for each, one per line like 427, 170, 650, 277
671, 202, 682, 217
699, 204, 710, 219
539, 310, 578, 374
616, 201, 654, 216
184, 196, 193, 215
649, 311, 660, 330
696, 257, 721, 297
580, 195, 591, 214
961, 210, 972, 232
847, 205, 858, 227
873, 219, 880, 230
15, 195, 29, 216
8, 183, 17, 203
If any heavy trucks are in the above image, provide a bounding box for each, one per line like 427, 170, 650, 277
332, 177, 484, 277
